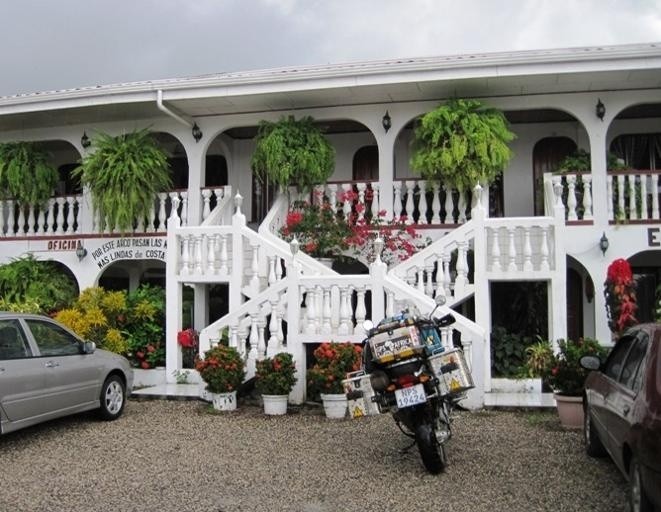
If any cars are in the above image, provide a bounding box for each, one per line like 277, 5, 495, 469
576, 322, 660, 511
0, 310, 133, 436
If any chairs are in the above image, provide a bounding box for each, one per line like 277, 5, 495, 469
0, 327, 26, 357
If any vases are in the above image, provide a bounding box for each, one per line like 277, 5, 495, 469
211, 388, 238, 410
321, 393, 349, 420
261, 391, 288, 415
553, 393, 587, 430
313, 257, 334, 268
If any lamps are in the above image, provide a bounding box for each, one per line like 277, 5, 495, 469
600, 230, 609, 255
382, 110, 391, 133
191, 121, 202, 143
82, 131, 92, 148
594, 98, 606, 120
75, 240, 88, 261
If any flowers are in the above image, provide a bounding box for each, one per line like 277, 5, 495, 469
196, 341, 247, 391
547, 337, 610, 395
604, 261, 639, 331
279, 187, 426, 262
253, 353, 298, 395
308, 344, 362, 393
179, 326, 200, 347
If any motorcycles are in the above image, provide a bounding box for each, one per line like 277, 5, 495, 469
341, 295, 474, 474
